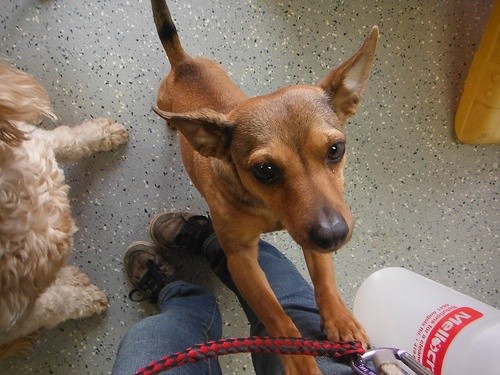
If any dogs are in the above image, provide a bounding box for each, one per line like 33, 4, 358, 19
0, 58, 129, 360
149, 0, 377, 375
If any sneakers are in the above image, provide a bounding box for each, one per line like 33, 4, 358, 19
149, 211, 214, 254
123, 241, 182, 307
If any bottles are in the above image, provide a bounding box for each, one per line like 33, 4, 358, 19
352, 266, 499, 375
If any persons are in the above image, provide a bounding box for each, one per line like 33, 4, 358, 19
111, 211, 377, 375
